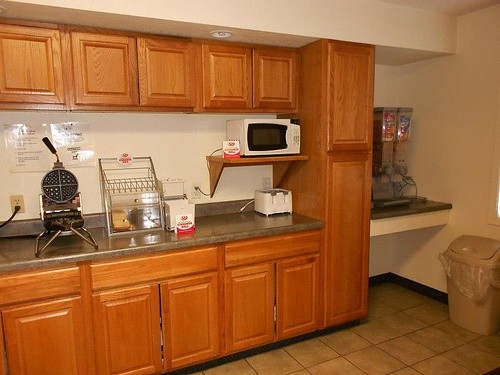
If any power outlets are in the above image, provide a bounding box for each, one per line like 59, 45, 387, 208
11, 195, 25, 213
191, 183, 202, 199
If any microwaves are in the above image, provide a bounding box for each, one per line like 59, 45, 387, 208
226, 118, 301, 156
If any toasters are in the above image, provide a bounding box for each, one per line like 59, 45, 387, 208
253, 188, 293, 216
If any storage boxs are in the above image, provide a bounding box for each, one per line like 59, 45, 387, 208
223, 140, 240, 159
164, 199, 195, 231
175, 214, 195, 235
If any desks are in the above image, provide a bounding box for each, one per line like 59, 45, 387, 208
370, 196, 453, 237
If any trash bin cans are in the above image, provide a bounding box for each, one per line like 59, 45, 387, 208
444, 235, 500, 336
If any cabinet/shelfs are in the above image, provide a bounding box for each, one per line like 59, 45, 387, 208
1, 262, 87, 375
85, 243, 221, 375
222, 229, 321, 364
65, 23, 199, 113
98, 156, 164, 236
0, 17, 71, 110
198, 38, 300, 112
272, 38, 375, 335
